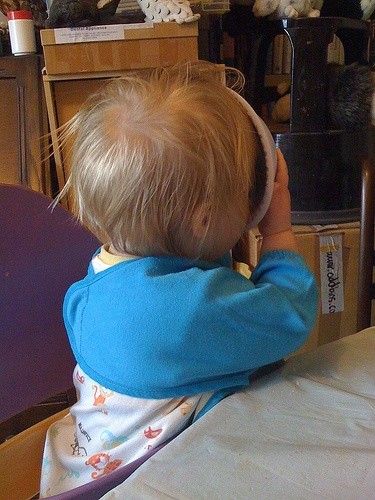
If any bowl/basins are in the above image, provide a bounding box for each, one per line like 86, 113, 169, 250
225, 87, 277, 230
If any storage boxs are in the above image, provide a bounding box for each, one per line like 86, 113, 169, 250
233, 221, 361, 362
40, 21, 199, 76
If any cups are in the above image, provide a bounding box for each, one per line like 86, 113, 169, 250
8, 11, 37, 56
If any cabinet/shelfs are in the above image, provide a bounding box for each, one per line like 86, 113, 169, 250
0, 15, 368, 207
0, 0, 375, 210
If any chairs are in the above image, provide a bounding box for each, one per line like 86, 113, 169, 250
0, 183, 103, 423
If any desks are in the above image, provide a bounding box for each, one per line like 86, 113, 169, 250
98, 325, 375, 500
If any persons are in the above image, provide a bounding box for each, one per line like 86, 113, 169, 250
38, 72, 318, 500
